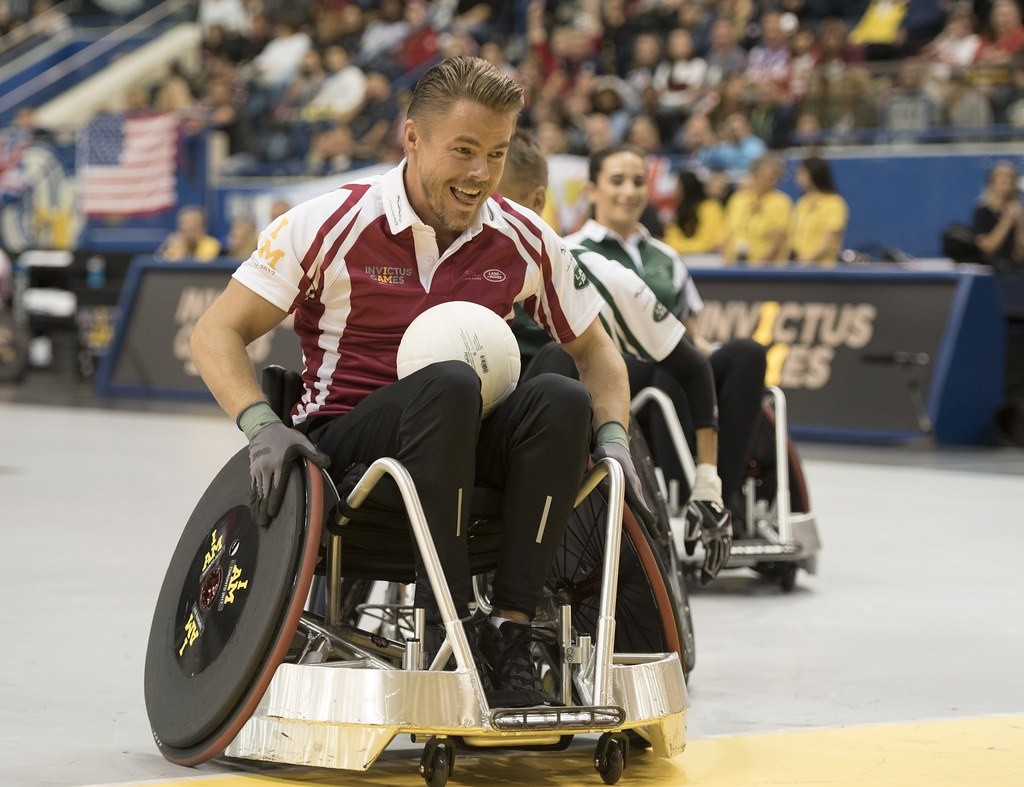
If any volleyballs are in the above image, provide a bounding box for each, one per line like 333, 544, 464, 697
398, 302, 522, 418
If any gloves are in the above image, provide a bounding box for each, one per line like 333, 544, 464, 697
236, 401, 332, 526
593, 422, 662, 539
687, 463, 725, 513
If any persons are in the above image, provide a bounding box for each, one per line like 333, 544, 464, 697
566, 145, 778, 537
0, 1, 1024, 443
188, 56, 733, 710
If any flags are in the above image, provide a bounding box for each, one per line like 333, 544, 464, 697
76, 107, 179, 219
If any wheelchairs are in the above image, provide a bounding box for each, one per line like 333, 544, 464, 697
144, 364, 695, 787
628, 384, 822, 592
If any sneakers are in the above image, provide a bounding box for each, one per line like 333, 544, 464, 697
476, 617, 557, 705
418, 616, 493, 707
